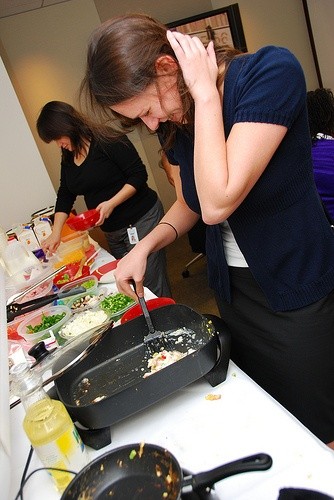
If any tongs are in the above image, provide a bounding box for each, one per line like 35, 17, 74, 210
6, 286, 86, 322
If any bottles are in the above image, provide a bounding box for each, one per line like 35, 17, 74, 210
2, 214, 53, 275
9, 362, 91, 496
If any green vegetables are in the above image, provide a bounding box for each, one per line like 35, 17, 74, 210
26, 312, 68, 346
57, 273, 69, 284
81, 280, 95, 290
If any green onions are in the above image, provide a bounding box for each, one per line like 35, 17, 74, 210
101, 293, 135, 321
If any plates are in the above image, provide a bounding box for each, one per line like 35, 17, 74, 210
66, 258, 96, 269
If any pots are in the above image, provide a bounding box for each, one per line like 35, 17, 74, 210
52, 303, 231, 450
59, 441, 272, 500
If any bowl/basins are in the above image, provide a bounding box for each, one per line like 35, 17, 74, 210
66, 209, 100, 232
67, 286, 109, 314
45, 236, 88, 268
94, 290, 137, 323
91, 259, 121, 284
18, 305, 72, 346
121, 297, 176, 325
5, 263, 53, 304
7, 304, 52, 340
54, 266, 90, 294
59, 307, 112, 341
59, 275, 99, 304
59, 230, 91, 253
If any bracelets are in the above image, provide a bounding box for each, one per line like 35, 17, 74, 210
160, 222, 178, 240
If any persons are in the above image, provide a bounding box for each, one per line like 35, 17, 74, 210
85, 13, 334, 444
37, 101, 173, 299
306, 88, 334, 224
132, 236, 135, 241
